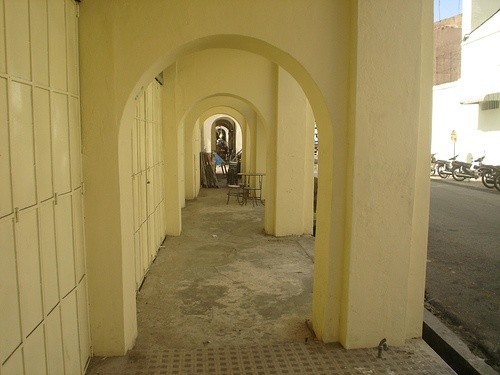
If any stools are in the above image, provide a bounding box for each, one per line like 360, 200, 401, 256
227, 184, 258, 208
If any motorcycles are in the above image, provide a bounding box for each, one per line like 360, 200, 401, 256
451, 151, 489, 181
483, 165, 500, 191
430, 153, 460, 178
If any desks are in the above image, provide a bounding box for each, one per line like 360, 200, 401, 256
237, 173, 266, 206
